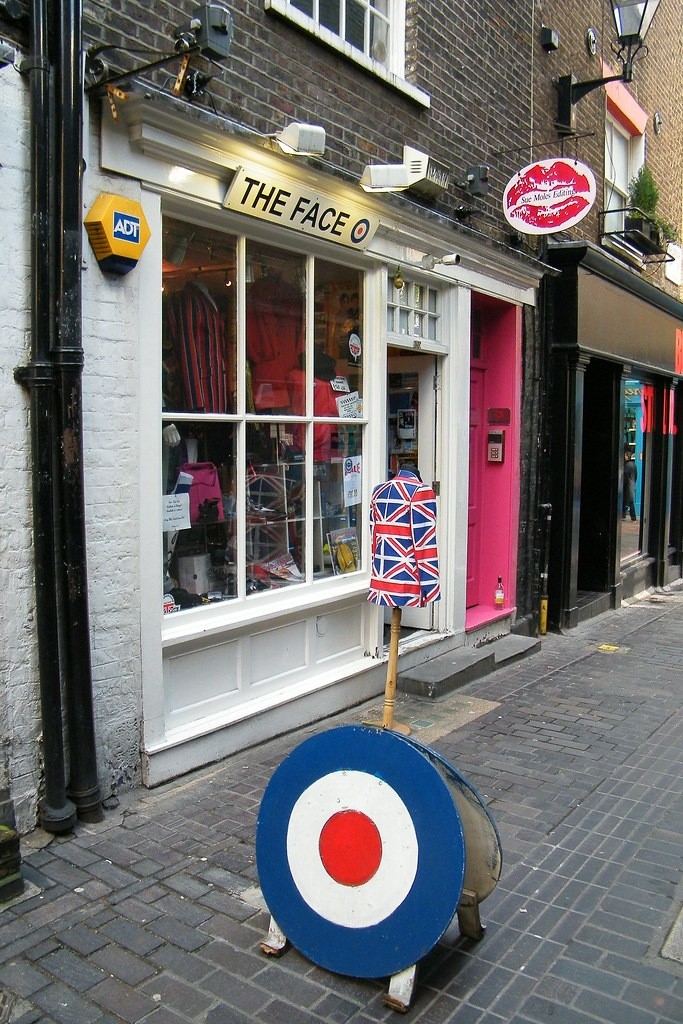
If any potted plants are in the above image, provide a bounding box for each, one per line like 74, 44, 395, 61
623, 163, 678, 255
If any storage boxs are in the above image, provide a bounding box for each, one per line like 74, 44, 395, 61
179, 552, 212, 596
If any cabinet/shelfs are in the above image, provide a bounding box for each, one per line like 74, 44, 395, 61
389, 387, 418, 455
249, 462, 351, 580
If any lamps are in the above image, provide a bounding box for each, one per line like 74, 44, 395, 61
263, 123, 326, 158
558, 0, 661, 137
85, 2, 233, 99
359, 164, 411, 194
455, 166, 490, 195
